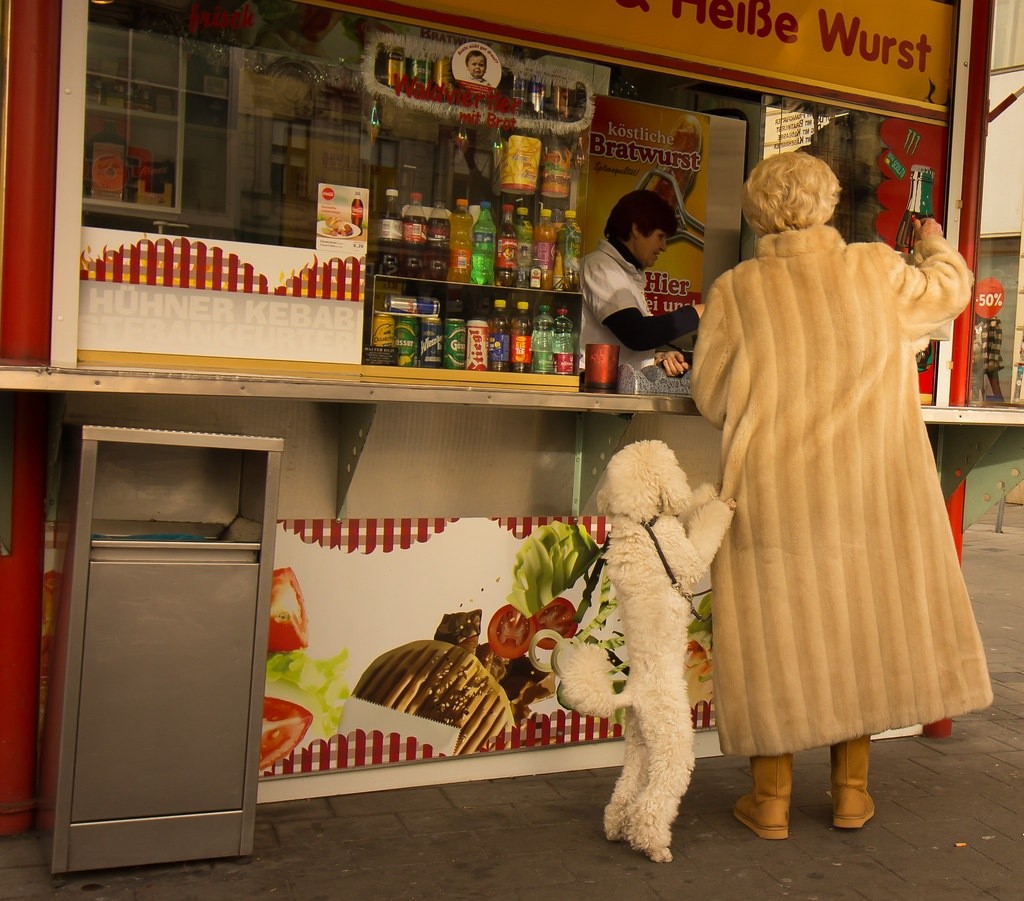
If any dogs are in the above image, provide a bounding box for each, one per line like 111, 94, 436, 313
555, 437, 737, 860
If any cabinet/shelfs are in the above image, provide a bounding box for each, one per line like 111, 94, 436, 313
87, 18, 584, 390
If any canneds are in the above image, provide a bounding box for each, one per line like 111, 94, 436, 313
372, 293, 489, 371
386, 47, 571, 118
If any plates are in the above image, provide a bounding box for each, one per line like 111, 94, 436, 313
316, 221, 361, 239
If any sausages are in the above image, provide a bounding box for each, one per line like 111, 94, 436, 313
330, 224, 353, 236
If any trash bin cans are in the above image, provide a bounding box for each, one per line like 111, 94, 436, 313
42, 419, 287, 872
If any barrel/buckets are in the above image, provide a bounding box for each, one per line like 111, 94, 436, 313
493, 135, 574, 198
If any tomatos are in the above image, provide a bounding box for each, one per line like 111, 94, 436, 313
268, 567, 309, 651
488, 597, 579, 660
259, 698, 312, 772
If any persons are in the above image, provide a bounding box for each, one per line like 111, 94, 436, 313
970, 316, 1005, 404
689, 151, 975, 840
577, 190, 706, 392
465, 50, 489, 85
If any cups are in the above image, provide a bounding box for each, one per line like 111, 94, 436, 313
584, 342, 620, 389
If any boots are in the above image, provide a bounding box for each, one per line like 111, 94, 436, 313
829, 734, 876, 830
732, 753, 793, 839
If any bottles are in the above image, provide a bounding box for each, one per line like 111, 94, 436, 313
374, 190, 580, 292
554, 309, 574, 375
511, 301, 533, 372
893, 163, 934, 371
487, 299, 510, 371
350, 192, 363, 236
88, 119, 124, 200
532, 305, 555, 374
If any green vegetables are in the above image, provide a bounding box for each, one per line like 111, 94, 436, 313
506, 521, 602, 617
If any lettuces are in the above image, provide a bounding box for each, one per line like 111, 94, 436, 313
265, 648, 350, 739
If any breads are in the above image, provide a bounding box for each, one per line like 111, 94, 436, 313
325, 216, 342, 229
351, 639, 515, 755
